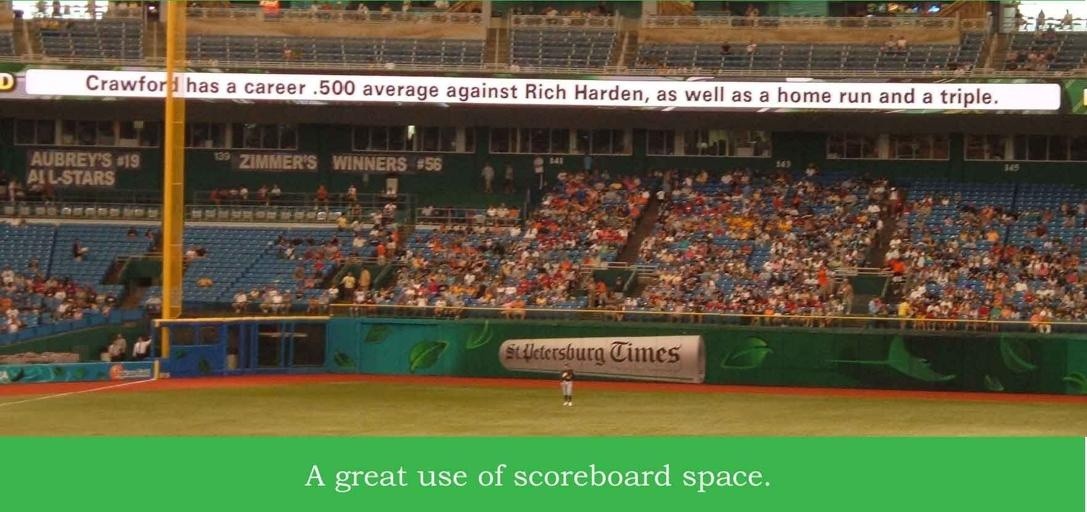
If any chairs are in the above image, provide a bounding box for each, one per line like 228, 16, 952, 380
0, 180, 1085, 342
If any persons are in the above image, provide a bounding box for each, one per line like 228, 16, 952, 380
401, 0, 412, 21
364, 55, 377, 72
282, 47, 291, 60
378, 2, 392, 18
997, 40, 1056, 71
745, 3, 758, 26
187, 2, 203, 19
489, 3, 605, 27
945, 57, 958, 71
35, 2, 47, 16
321, 0, 333, 21
509, 59, 520, 74
867, 3, 937, 24
382, 59, 395, 70
719, 40, 732, 56
1034, 10, 1045, 29
1055, 9, 1072, 30
1012, 9, 1028, 31
558, 361, 573, 407
0, 153, 1085, 363
432, 0, 450, 10
355, 3, 371, 23
884, 34, 896, 56
894, 36, 907, 60
743, 40, 753, 56
308, 2, 321, 25
51, 1, 62, 14
331, 2, 347, 21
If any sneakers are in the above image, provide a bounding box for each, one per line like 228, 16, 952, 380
563, 401, 572, 406
